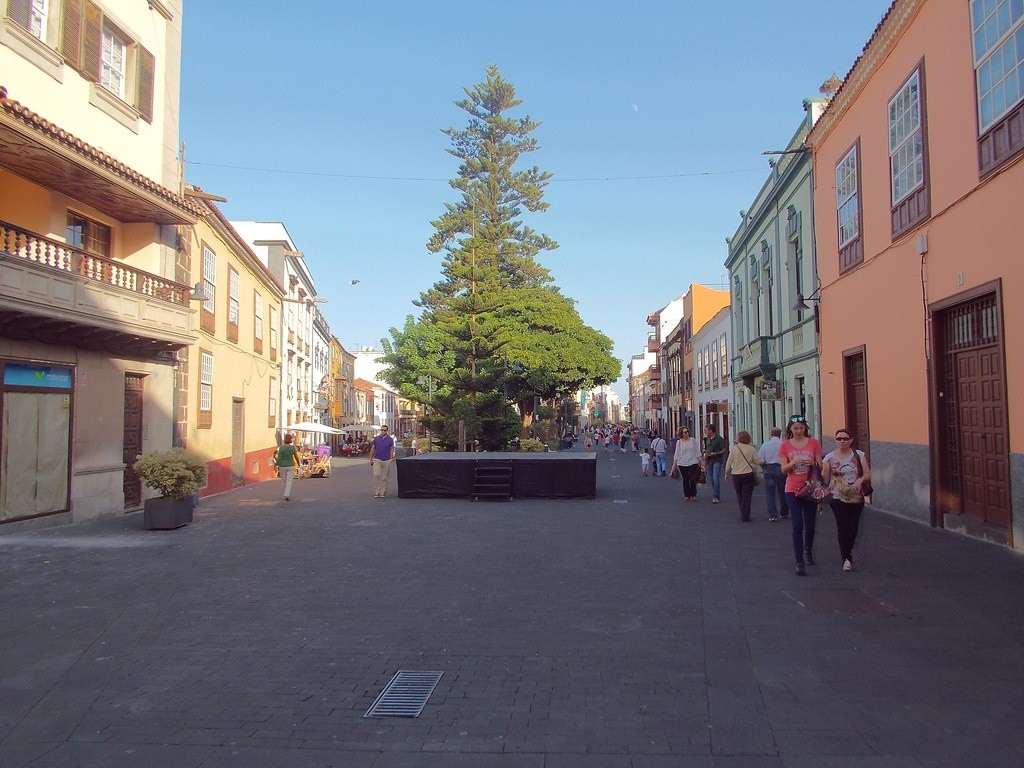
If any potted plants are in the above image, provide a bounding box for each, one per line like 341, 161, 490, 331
132, 446, 208, 530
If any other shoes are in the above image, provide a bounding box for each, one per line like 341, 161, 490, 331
802, 545, 815, 565
683, 495, 696, 502
791, 562, 808, 578
780, 512, 791, 519
662, 470, 666, 477
373, 491, 387, 498
768, 514, 778, 522
283, 495, 291, 501
712, 497, 720, 504
741, 514, 750, 523
842, 560, 853, 572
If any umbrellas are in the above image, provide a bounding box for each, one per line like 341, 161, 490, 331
277, 421, 345, 480
341, 424, 381, 437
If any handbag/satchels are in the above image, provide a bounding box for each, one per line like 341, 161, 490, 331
668, 465, 680, 479
795, 461, 831, 504
697, 465, 706, 486
852, 449, 874, 495
752, 470, 761, 486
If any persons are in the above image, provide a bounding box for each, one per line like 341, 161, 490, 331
725, 431, 758, 522
594, 423, 663, 453
275, 434, 300, 501
671, 426, 705, 500
412, 437, 423, 456
370, 425, 397, 498
758, 428, 790, 521
704, 425, 726, 503
821, 429, 871, 572
584, 434, 592, 452
779, 415, 824, 575
640, 448, 650, 476
651, 435, 667, 476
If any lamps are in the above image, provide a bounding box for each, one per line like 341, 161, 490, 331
792, 288, 819, 312
190, 282, 210, 301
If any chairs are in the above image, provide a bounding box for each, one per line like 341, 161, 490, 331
276, 454, 333, 479
336, 444, 369, 457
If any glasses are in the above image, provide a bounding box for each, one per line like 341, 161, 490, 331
791, 414, 806, 423
685, 430, 690, 434
836, 435, 852, 444
380, 429, 387, 431
